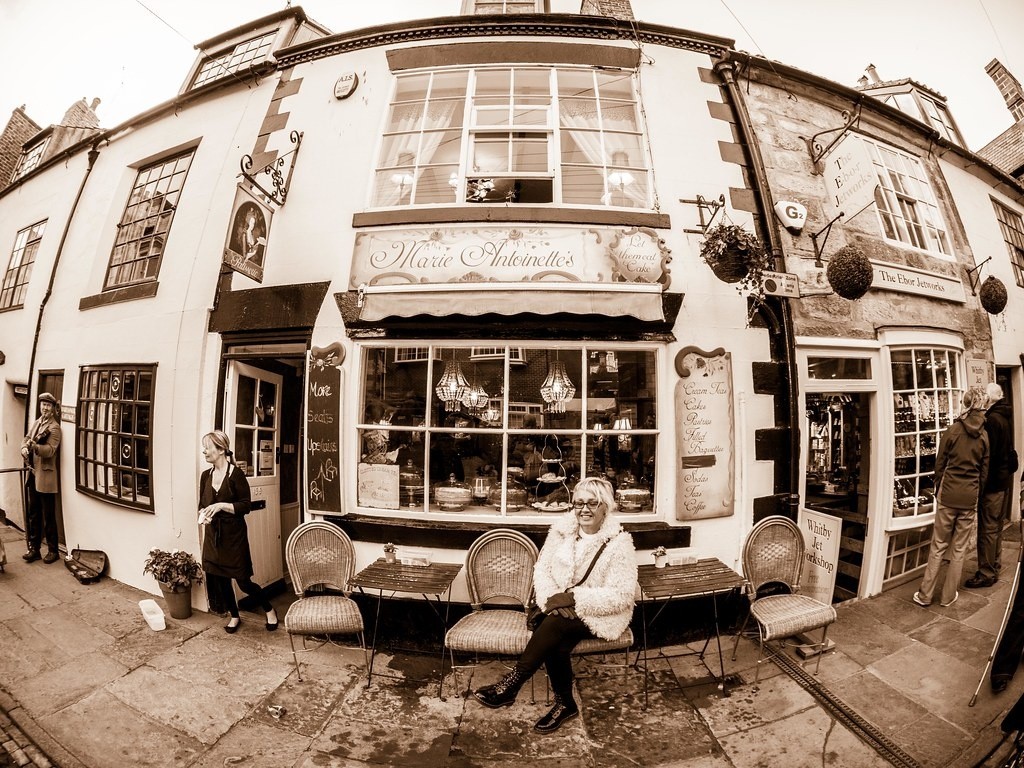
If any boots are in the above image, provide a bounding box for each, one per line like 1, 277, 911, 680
534, 693, 580, 734
473, 666, 532, 709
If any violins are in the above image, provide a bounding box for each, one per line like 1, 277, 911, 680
20, 428, 52, 460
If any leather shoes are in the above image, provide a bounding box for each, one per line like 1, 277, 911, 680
43, 552, 60, 563
23, 549, 42, 561
965, 570, 997, 588
992, 679, 1007, 692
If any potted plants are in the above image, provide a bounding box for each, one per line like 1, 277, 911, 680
383, 542, 398, 563
650, 546, 669, 568
697, 222, 770, 329
142, 547, 203, 619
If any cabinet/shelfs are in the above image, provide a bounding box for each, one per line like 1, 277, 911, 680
809, 393, 945, 508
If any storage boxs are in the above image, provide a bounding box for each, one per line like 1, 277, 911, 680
664, 546, 699, 567
399, 551, 433, 566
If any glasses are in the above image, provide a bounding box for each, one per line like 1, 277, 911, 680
574, 502, 602, 509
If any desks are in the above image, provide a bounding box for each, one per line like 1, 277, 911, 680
347, 557, 464, 700
633, 558, 751, 711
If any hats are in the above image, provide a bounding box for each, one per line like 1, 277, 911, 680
39, 392, 56, 403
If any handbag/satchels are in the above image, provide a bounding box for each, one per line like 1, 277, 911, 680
527, 585, 548, 631
1008, 449, 1018, 476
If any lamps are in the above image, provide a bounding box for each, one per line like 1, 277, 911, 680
391, 150, 414, 205
607, 149, 636, 209
436, 347, 633, 445
448, 159, 495, 202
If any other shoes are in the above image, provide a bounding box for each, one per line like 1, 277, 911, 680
266, 609, 278, 631
226, 617, 241, 633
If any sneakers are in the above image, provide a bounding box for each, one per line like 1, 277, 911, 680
940, 591, 959, 606
911, 590, 929, 605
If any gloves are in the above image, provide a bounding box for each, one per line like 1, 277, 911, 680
545, 592, 578, 619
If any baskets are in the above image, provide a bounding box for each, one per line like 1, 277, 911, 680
706, 249, 749, 283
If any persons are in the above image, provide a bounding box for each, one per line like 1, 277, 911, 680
913, 387, 990, 607
472, 478, 638, 734
963, 383, 1015, 588
197, 430, 278, 634
20, 392, 61, 564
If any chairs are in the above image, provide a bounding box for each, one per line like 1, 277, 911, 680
284, 520, 370, 683
732, 515, 837, 693
444, 527, 634, 707
